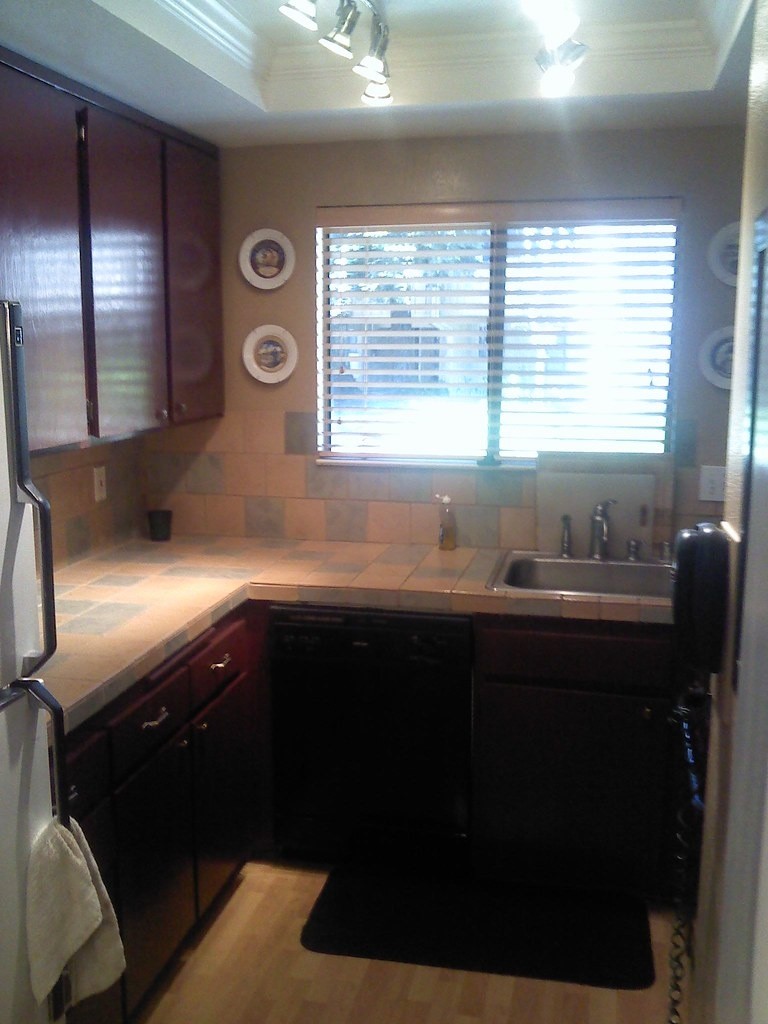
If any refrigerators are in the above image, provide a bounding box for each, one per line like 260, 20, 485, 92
0, 298, 66, 1024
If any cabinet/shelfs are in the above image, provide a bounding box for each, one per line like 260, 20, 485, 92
51, 616, 261, 1024
472, 626, 673, 892
0, 44, 224, 454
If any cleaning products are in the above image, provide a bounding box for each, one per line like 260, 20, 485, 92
435, 493, 457, 551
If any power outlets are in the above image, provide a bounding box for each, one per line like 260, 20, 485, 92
701, 465, 725, 501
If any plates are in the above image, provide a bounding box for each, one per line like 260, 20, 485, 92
707, 221, 740, 287
242, 324, 298, 383
239, 228, 295, 289
697, 325, 734, 390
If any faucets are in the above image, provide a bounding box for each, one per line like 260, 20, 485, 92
586, 498, 618, 562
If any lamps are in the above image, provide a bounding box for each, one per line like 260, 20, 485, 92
536, 39, 591, 74
360, 81, 393, 107
278, 0, 319, 32
318, 1, 359, 59
351, 15, 389, 85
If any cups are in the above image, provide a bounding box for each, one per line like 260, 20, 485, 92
147, 509, 173, 540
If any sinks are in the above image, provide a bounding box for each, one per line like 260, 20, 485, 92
483, 548, 677, 599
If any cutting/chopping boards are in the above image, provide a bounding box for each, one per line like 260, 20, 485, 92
538, 472, 654, 561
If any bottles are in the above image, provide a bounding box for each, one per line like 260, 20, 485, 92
660, 539, 673, 563
434, 493, 457, 551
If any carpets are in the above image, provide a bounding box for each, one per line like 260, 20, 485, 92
299, 869, 656, 991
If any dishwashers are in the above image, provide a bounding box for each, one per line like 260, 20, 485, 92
266, 606, 474, 876
